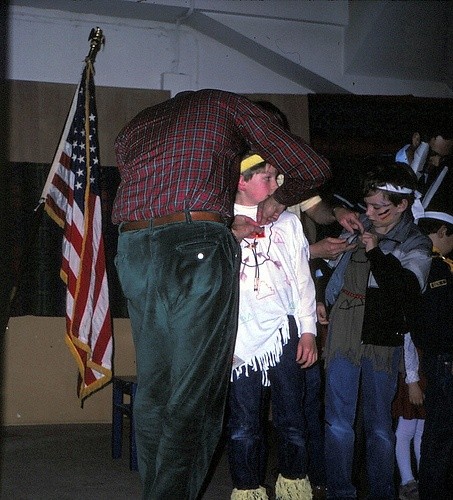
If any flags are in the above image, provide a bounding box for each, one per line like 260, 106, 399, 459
39, 60, 114, 409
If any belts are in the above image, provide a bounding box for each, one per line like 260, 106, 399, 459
120, 212, 226, 232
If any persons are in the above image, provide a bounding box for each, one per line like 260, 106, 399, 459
228, 102, 453, 500
111, 88, 332, 500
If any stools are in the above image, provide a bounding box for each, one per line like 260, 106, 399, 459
113, 375, 137, 470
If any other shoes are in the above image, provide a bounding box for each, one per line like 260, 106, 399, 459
275, 473, 313, 500
230, 485, 268, 500
399, 481, 418, 500
